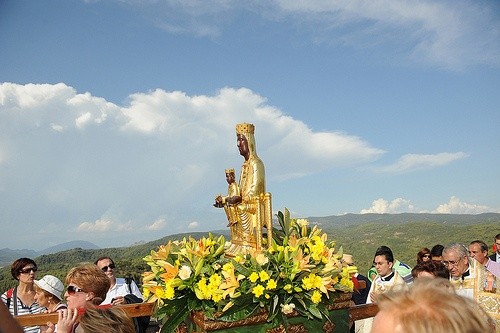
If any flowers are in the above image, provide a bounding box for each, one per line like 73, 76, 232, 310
140, 208, 357, 333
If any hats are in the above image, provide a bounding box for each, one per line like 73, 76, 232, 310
430, 245, 445, 256
33, 275, 65, 300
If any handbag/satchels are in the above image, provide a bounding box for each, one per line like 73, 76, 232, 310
126, 277, 150, 333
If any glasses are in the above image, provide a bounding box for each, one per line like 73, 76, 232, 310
423, 254, 432, 259
102, 263, 116, 272
20, 266, 37, 274
443, 254, 465, 267
65, 285, 85, 296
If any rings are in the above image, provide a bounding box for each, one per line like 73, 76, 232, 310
63, 316, 67, 319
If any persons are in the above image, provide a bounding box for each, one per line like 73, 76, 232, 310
216, 168, 242, 227
366, 250, 407, 304
442, 242, 500, 333
468, 240, 500, 279
94, 257, 144, 333
489, 234, 500, 264
2, 258, 47, 333
212, 123, 265, 255
410, 259, 450, 289
414, 246, 432, 263
368, 246, 412, 283
347, 263, 371, 305
34, 275, 68, 333
371, 278, 495, 333
430, 244, 446, 261
75, 308, 135, 333
40, 262, 111, 333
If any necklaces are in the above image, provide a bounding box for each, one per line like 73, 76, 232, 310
53, 301, 61, 310
